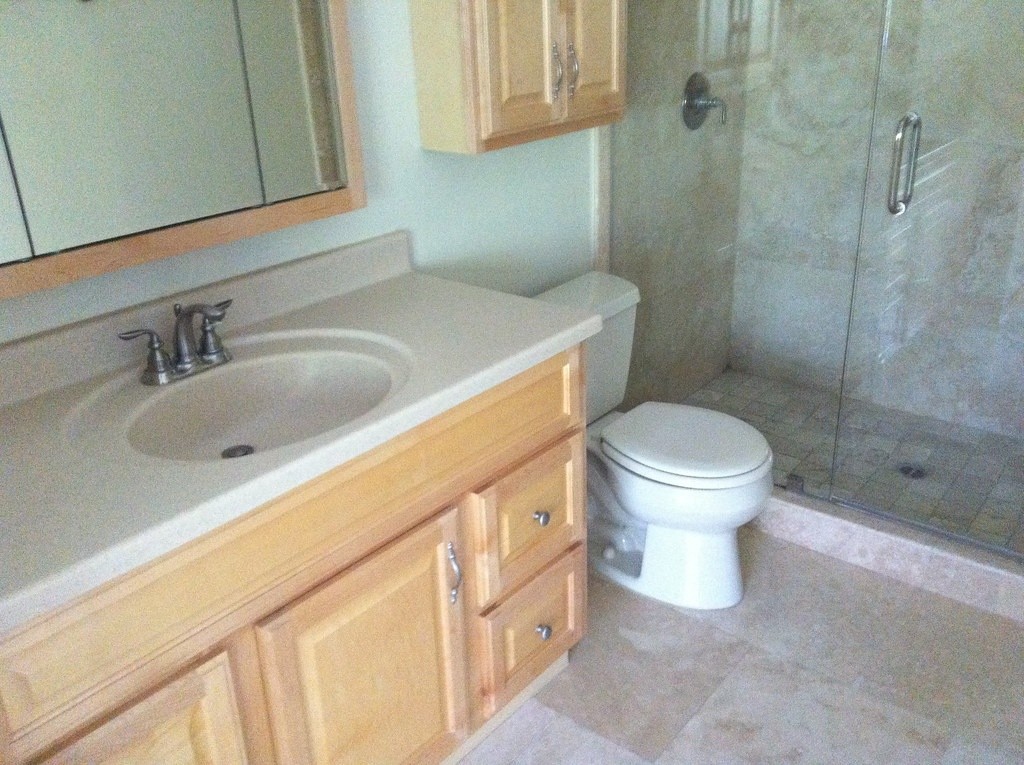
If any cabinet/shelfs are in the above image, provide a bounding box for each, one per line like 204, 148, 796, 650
409, 0, 627, 155
470, 424, 588, 717
29, 504, 478, 765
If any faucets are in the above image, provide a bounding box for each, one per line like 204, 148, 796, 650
173, 302, 224, 374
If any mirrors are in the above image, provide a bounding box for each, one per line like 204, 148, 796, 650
0, 0, 368, 301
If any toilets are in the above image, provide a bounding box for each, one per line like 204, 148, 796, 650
513, 271, 773, 609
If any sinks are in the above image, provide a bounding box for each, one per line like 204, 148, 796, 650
55, 326, 415, 486
